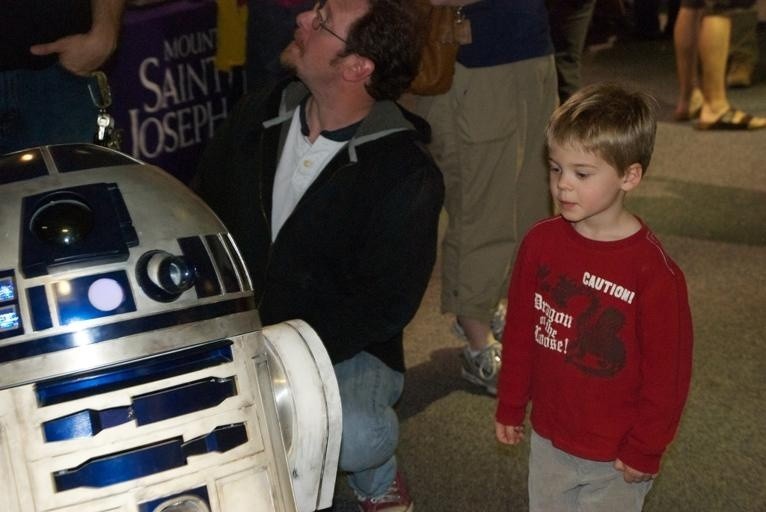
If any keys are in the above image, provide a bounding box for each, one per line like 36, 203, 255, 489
95, 104, 124, 152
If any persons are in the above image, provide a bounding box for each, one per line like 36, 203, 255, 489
670, 3, 766, 133
0, 1, 129, 156
544, 1, 762, 102
403, 0, 566, 402
188, 1, 450, 509
492, 83, 697, 510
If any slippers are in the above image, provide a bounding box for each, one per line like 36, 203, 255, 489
694, 107, 766, 131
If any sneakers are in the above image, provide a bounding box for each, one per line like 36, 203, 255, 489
350, 468, 417, 512
725, 59, 753, 88
446, 298, 506, 397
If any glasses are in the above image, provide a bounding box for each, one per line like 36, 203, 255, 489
312, 1, 349, 44
